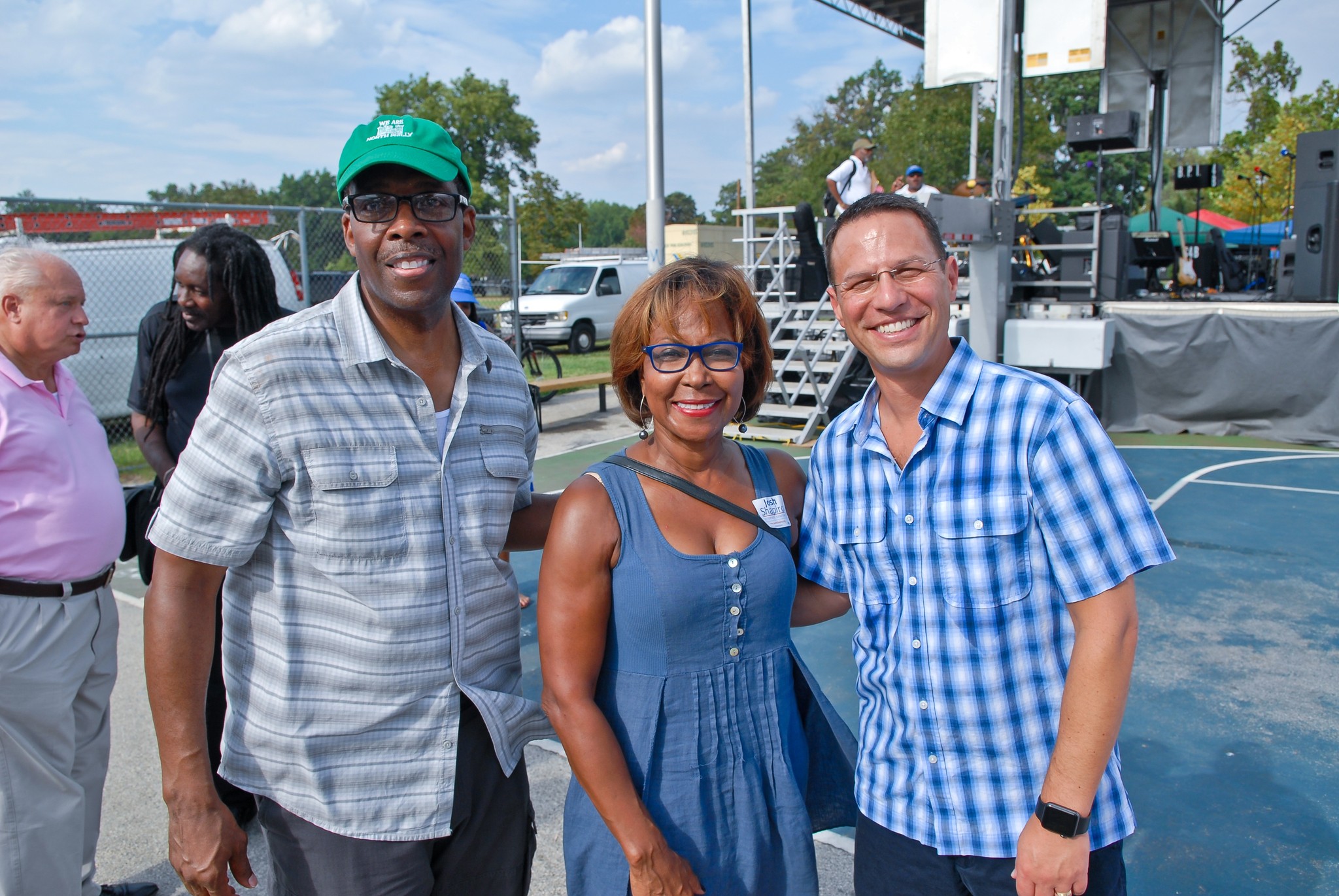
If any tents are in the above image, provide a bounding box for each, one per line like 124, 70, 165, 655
1126, 204, 1294, 248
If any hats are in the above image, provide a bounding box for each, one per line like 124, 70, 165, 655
905, 165, 924, 176
852, 138, 877, 153
336, 114, 473, 207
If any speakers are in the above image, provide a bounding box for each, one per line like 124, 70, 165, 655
1066, 109, 1140, 151
1058, 228, 1130, 301
1292, 128, 1339, 302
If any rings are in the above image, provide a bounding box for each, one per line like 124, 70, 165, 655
1055, 890, 1071, 896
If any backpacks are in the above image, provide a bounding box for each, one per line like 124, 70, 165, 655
823, 159, 856, 217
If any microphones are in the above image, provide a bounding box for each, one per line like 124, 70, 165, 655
1279, 148, 1295, 159
1254, 166, 1272, 178
1237, 175, 1252, 180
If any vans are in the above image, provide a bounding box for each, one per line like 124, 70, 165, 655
0, 237, 304, 422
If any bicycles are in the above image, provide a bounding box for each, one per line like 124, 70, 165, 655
477, 309, 563, 401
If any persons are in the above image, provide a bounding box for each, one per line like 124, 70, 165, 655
0, 249, 157, 896
450, 273, 534, 609
826, 138, 878, 223
125, 223, 299, 822
140, 114, 563, 896
784, 192, 1176, 896
890, 165, 940, 208
537, 255, 856, 896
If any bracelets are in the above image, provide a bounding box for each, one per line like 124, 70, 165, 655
163, 466, 176, 486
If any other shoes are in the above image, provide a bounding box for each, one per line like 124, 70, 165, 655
208, 755, 258, 825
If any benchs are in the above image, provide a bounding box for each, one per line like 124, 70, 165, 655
528, 372, 613, 433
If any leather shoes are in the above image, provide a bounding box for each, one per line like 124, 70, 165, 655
99, 882, 158, 896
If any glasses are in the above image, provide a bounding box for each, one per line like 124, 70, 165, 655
341, 190, 468, 223
641, 341, 743, 373
832, 255, 945, 296
909, 171, 922, 178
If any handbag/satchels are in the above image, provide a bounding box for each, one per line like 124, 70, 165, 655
788, 648, 860, 833
119, 481, 153, 564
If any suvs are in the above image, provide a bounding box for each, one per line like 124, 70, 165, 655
497, 255, 649, 355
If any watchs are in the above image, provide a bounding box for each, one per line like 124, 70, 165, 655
1035, 796, 1092, 838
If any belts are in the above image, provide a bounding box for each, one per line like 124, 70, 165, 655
0, 562, 115, 597
